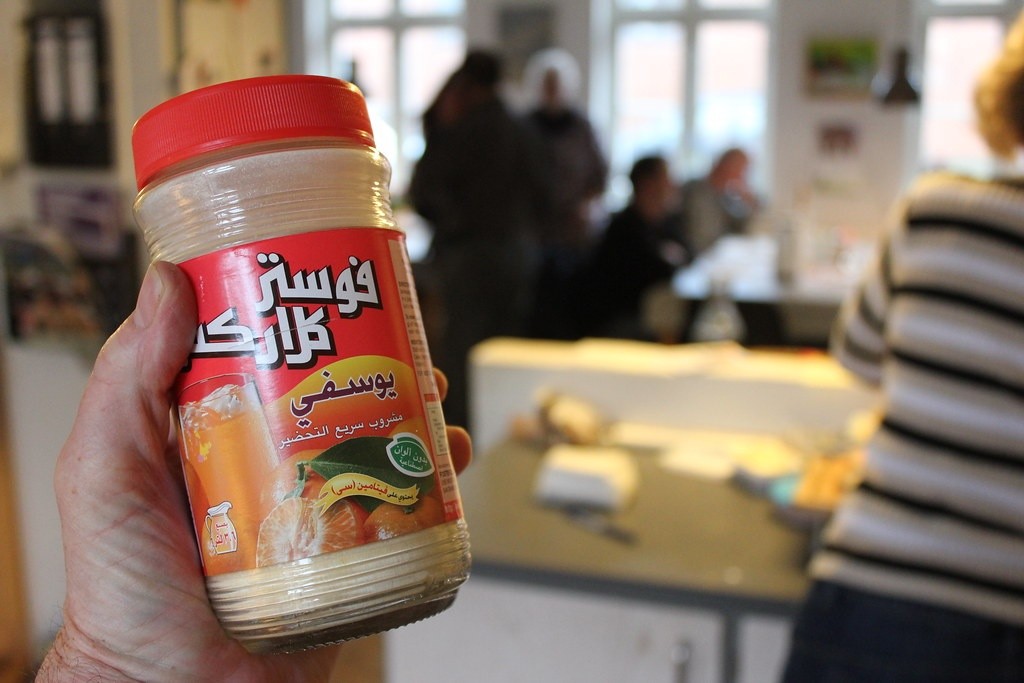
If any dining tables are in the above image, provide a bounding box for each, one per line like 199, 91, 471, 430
671, 233, 878, 352
456, 417, 823, 683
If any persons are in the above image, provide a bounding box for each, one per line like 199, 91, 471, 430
778, 3, 1024, 683
32, 260, 471, 683
592, 149, 784, 347
408, 48, 608, 430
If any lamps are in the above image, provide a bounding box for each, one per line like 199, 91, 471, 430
871, 1, 921, 106
